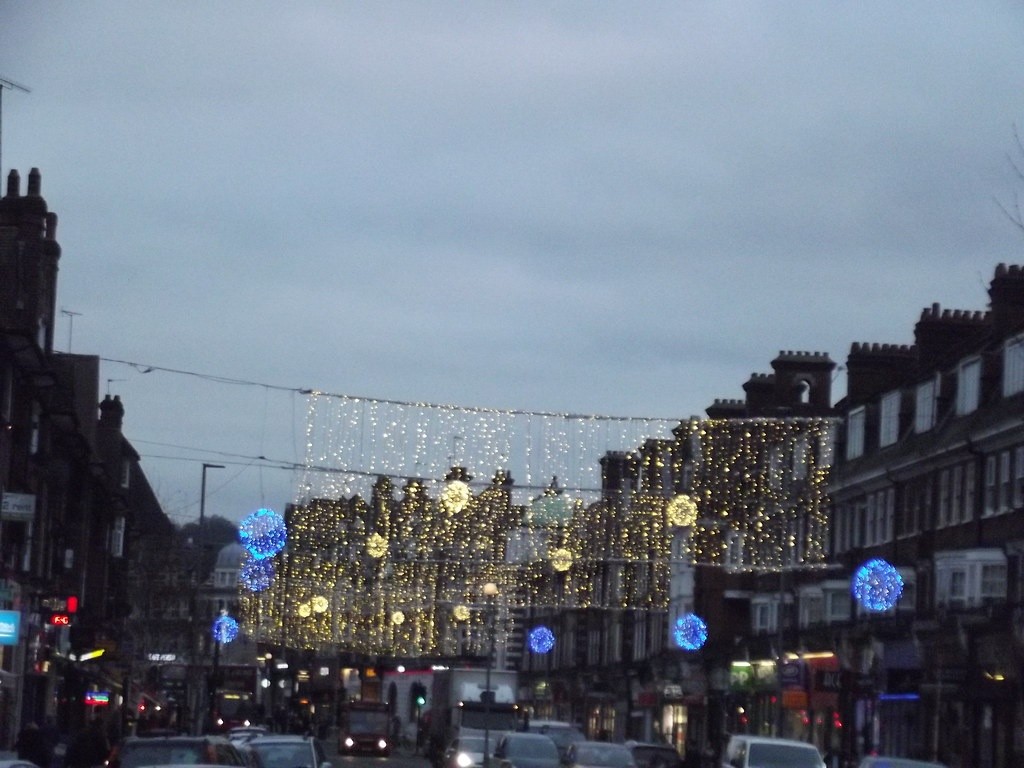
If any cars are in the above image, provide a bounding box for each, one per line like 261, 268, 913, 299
722, 736, 828, 768
443, 720, 685, 768
0, 726, 331, 768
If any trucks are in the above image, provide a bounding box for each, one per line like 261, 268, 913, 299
338, 700, 390, 751
429, 668, 516, 761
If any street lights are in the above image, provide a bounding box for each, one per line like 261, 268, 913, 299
188, 463, 224, 656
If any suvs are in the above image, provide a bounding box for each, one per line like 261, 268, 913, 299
135, 661, 209, 738
